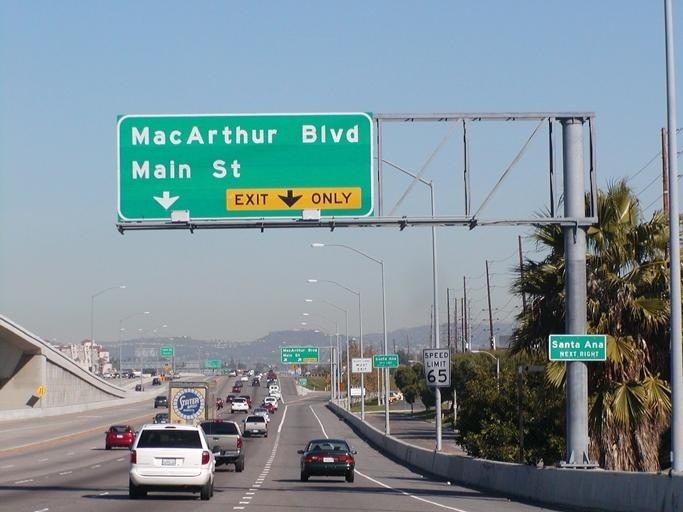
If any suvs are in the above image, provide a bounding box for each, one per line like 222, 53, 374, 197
195, 418, 245, 472
128, 420, 217, 499
240, 415, 270, 438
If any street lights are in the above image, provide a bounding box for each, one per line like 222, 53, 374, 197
299, 294, 352, 412
84, 281, 189, 394
270, 340, 292, 353
310, 242, 392, 435
306, 278, 367, 421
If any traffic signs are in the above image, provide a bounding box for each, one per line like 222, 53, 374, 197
546, 332, 610, 366
350, 357, 372, 372
373, 353, 399, 370
109, 109, 376, 226
280, 345, 320, 365
204, 358, 221, 369
418, 348, 455, 390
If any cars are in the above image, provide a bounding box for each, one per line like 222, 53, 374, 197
380, 387, 405, 404
153, 395, 167, 409
102, 424, 137, 451
150, 413, 169, 424
223, 365, 281, 422
215, 396, 224, 411
296, 438, 357, 482
